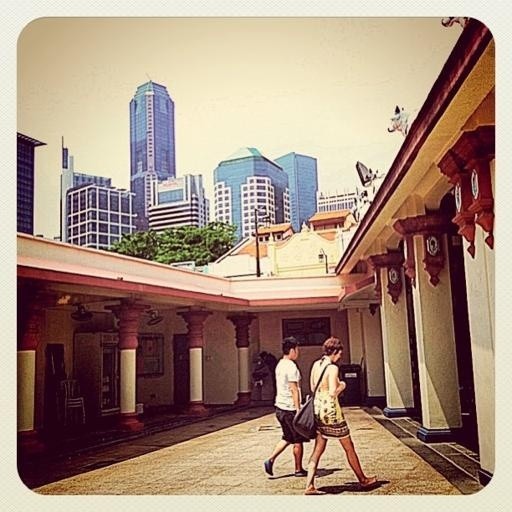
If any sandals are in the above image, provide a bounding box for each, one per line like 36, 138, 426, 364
305, 487, 328, 497
358, 477, 379, 489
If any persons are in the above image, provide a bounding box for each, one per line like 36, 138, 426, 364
304, 336, 378, 494
263, 334, 319, 477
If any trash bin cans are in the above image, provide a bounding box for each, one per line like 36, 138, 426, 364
340, 364, 362, 404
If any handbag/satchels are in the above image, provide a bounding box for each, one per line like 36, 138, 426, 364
292, 394, 318, 442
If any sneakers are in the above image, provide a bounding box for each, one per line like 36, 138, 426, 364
296, 469, 308, 478
264, 460, 274, 477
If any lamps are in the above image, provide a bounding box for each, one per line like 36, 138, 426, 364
70, 303, 93, 322
145, 308, 163, 325
